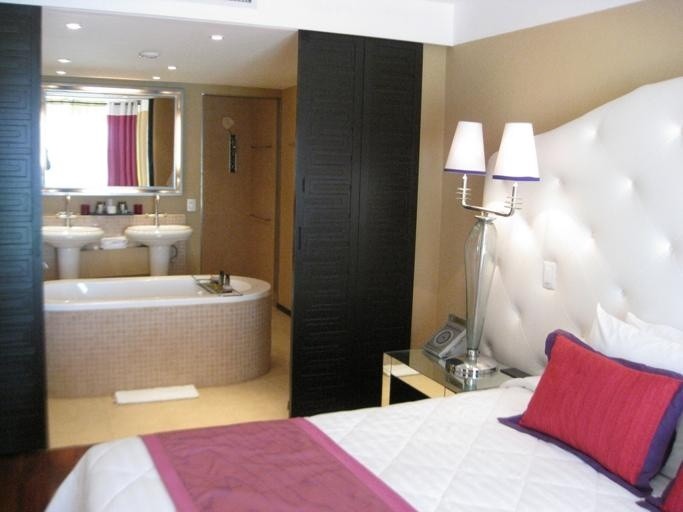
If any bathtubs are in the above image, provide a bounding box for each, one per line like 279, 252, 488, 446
43, 274, 273, 313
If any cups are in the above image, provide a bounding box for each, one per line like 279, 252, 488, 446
134, 204, 143, 215
81, 204, 90, 214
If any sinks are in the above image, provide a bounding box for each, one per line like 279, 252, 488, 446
41, 225, 104, 249
124, 224, 194, 246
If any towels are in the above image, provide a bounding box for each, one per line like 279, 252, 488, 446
114, 384, 199, 404
100, 235, 129, 250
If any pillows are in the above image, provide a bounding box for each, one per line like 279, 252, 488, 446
494, 304, 682, 509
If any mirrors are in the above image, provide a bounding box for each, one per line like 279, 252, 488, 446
38, 83, 184, 198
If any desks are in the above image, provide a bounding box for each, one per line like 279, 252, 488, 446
377, 350, 525, 407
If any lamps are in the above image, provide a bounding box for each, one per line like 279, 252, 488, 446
444, 112, 543, 386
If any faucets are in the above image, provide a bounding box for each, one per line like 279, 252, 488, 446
153, 193, 160, 225
65, 195, 70, 227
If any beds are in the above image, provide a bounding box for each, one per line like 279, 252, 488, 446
65, 74, 683, 512
